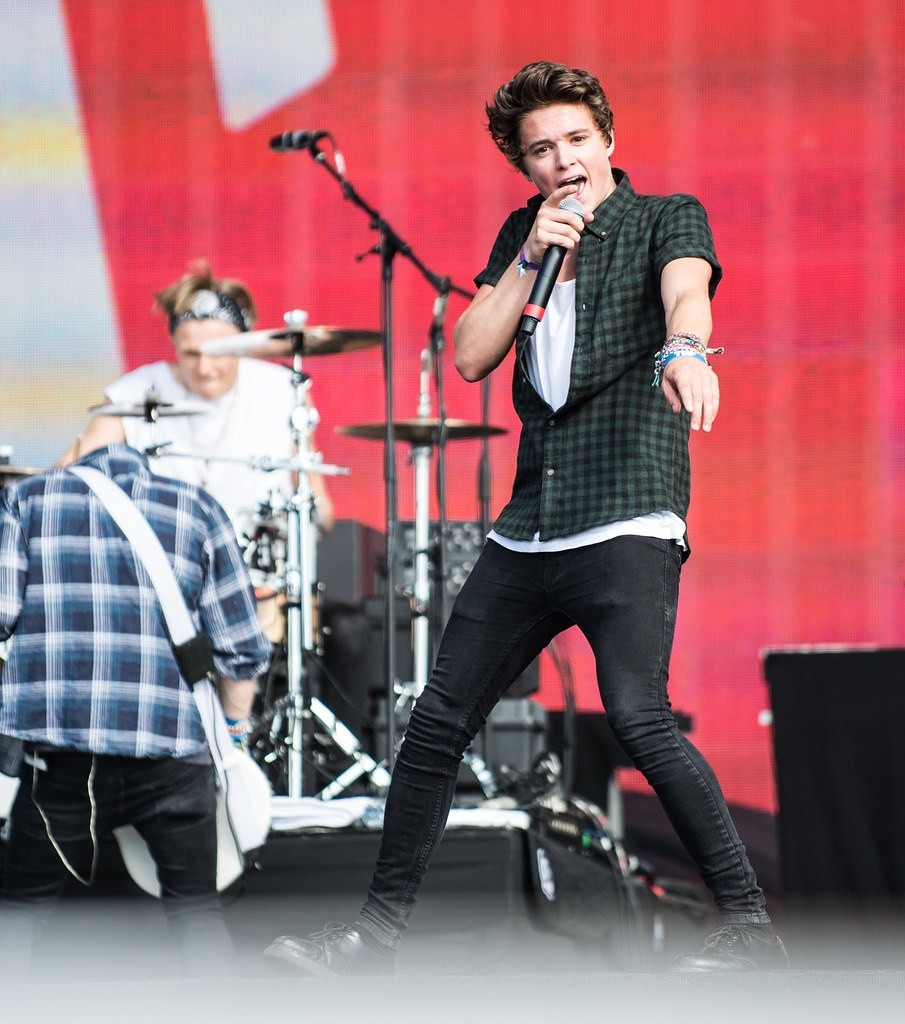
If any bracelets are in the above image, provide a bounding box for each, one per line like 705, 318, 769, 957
641, 333, 724, 386
515, 244, 542, 278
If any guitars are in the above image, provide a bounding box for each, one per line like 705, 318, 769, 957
112, 742, 277, 902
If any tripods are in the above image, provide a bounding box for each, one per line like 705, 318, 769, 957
236, 349, 496, 817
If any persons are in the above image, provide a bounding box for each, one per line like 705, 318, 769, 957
265, 61, 796, 989
61, 267, 334, 657
0, 426, 276, 955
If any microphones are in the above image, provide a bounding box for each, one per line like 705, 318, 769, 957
522, 199, 585, 339
270, 129, 327, 151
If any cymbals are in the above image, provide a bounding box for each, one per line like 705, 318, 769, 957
196, 323, 385, 359
333, 416, 511, 441
86, 397, 211, 419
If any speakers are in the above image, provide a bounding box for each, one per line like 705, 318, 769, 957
766, 647, 905, 897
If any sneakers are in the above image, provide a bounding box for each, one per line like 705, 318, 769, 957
672, 927, 791, 971
261, 924, 393, 977
